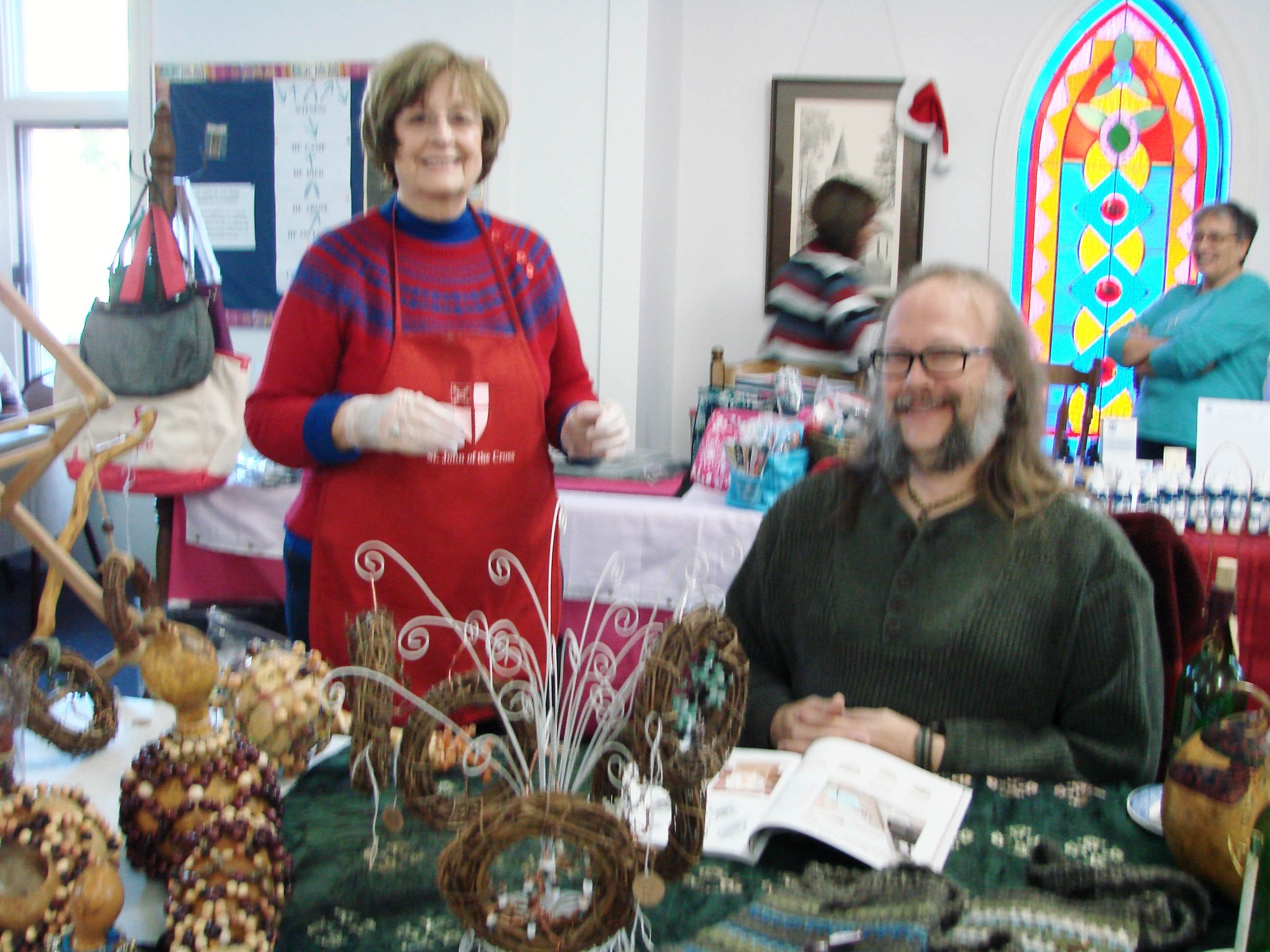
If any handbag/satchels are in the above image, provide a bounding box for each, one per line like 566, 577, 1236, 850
53, 172, 251, 495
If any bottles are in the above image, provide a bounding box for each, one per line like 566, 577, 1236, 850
709, 347, 726, 390
1055, 461, 1270, 536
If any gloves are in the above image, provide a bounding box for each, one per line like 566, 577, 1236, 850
562, 400, 634, 462
341, 386, 475, 456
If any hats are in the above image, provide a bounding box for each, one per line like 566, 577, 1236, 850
894, 73, 952, 176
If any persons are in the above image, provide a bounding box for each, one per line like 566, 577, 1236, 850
765, 180, 885, 365
1108, 203, 1270, 463
723, 263, 1164, 783
244, 40, 631, 739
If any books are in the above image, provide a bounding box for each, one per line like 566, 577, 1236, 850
618, 735, 974, 873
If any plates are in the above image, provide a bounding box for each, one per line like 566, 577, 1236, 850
1126, 783, 1163, 838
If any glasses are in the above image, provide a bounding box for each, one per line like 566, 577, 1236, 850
870, 342, 1002, 377
1190, 229, 1241, 242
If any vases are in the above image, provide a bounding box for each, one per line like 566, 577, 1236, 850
763, 74, 930, 316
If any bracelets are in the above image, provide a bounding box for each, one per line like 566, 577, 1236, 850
914, 726, 935, 772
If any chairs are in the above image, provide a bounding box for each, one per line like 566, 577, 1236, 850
1107, 513, 1209, 776
1029, 360, 1105, 462
708, 342, 868, 398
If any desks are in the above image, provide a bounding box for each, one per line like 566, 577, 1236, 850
2, 693, 1270, 952
171, 464, 1270, 725
